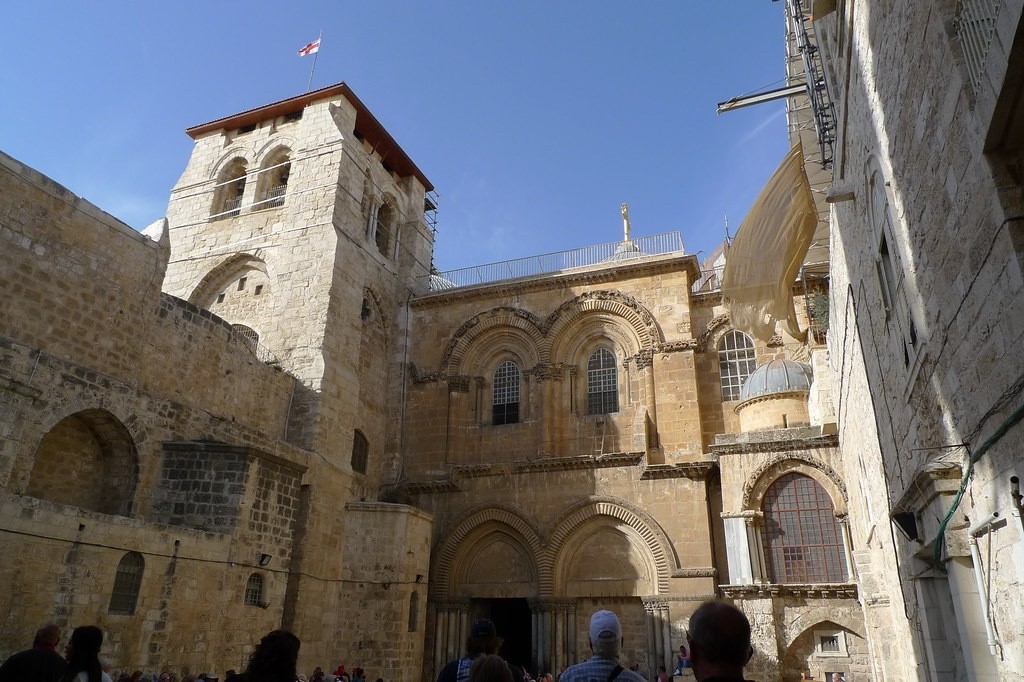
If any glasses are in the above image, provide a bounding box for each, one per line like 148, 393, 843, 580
160, 677, 169, 680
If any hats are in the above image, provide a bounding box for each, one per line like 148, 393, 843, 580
206, 673, 218, 679
588, 610, 622, 641
470, 619, 496, 638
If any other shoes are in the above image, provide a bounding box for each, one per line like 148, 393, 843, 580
677, 672, 683, 676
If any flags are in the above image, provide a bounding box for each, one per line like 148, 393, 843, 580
296, 39, 322, 57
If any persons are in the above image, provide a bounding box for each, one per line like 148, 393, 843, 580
0, 600, 845, 682
